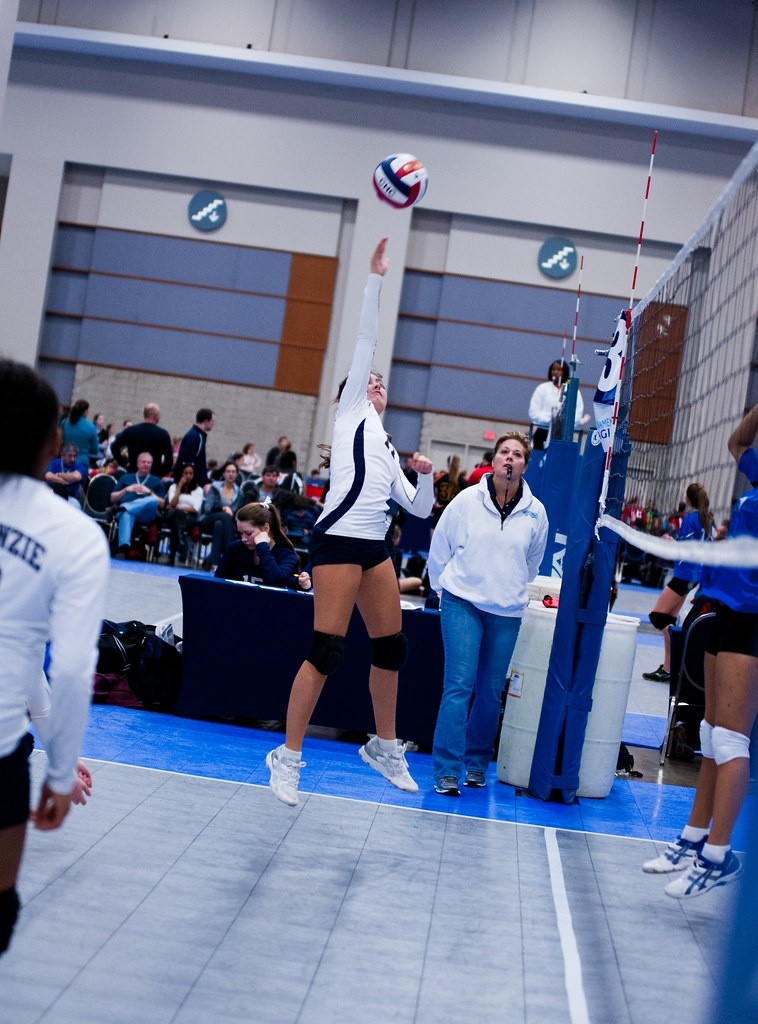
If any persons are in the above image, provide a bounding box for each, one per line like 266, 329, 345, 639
296, 562, 314, 593
0, 356, 110, 959
621, 495, 686, 540
110, 402, 173, 479
642, 483, 713, 681
172, 407, 215, 487
266, 239, 434, 806
105, 452, 166, 558
123, 420, 133, 428
167, 462, 244, 571
528, 358, 583, 450
90, 413, 116, 469
242, 465, 325, 534
45, 442, 84, 510
641, 403, 758, 899
265, 435, 298, 474
216, 442, 262, 481
62, 398, 98, 479
385, 451, 494, 593
207, 459, 217, 478
214, 497, 300, 588
427, 431, 549, 796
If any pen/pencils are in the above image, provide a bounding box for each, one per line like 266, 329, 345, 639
294, 574, 312, 579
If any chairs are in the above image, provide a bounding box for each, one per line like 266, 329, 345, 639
659, 608, 717, 765
78, 467, 256, 571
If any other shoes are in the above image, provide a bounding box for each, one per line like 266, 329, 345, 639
115, 544, 130, 559
167, 557, 175, 566
131, 549, 148, 562
201, 559, 213, 571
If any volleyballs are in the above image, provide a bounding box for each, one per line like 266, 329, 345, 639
373, 154, 428, 208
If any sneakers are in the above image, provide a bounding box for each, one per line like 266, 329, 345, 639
463, 770, 487, 787
433, 776, 461, 796
642, 664, 670, 683
664, 849, 745, 900
642, 835, 710, 873
267, 744, 307, 806
358, 735, 420, 793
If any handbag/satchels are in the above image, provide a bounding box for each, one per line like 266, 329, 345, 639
96, 621, 185, 714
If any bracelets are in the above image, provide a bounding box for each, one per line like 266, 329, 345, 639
57, 473, 59, 477
147, 489, 152, 495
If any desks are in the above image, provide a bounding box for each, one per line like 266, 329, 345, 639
179, 574, 446, 753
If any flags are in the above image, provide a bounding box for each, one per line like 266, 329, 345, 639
592, 311, 626, 453
